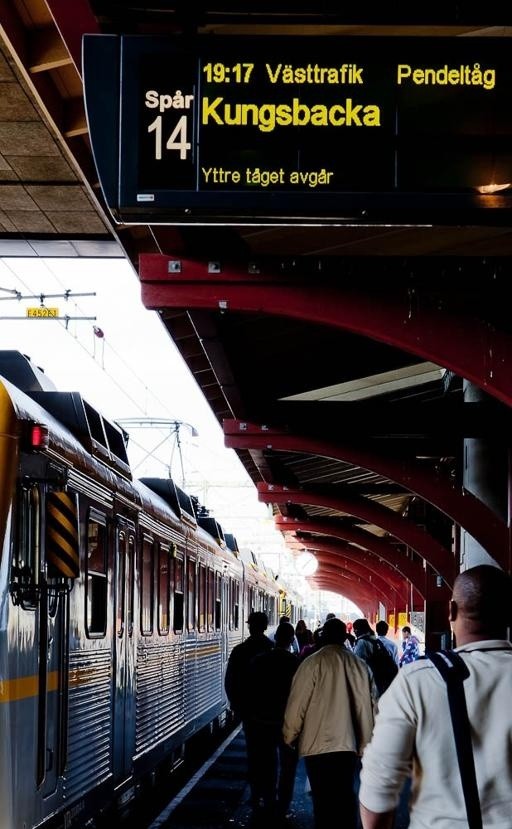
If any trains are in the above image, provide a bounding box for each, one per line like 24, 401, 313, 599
0, 341, 323, 829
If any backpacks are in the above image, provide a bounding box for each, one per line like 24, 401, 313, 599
355, 637, 398, 682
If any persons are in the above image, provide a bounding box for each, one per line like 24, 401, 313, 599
223, 609, 420, 829
358, 564, 512, 829
